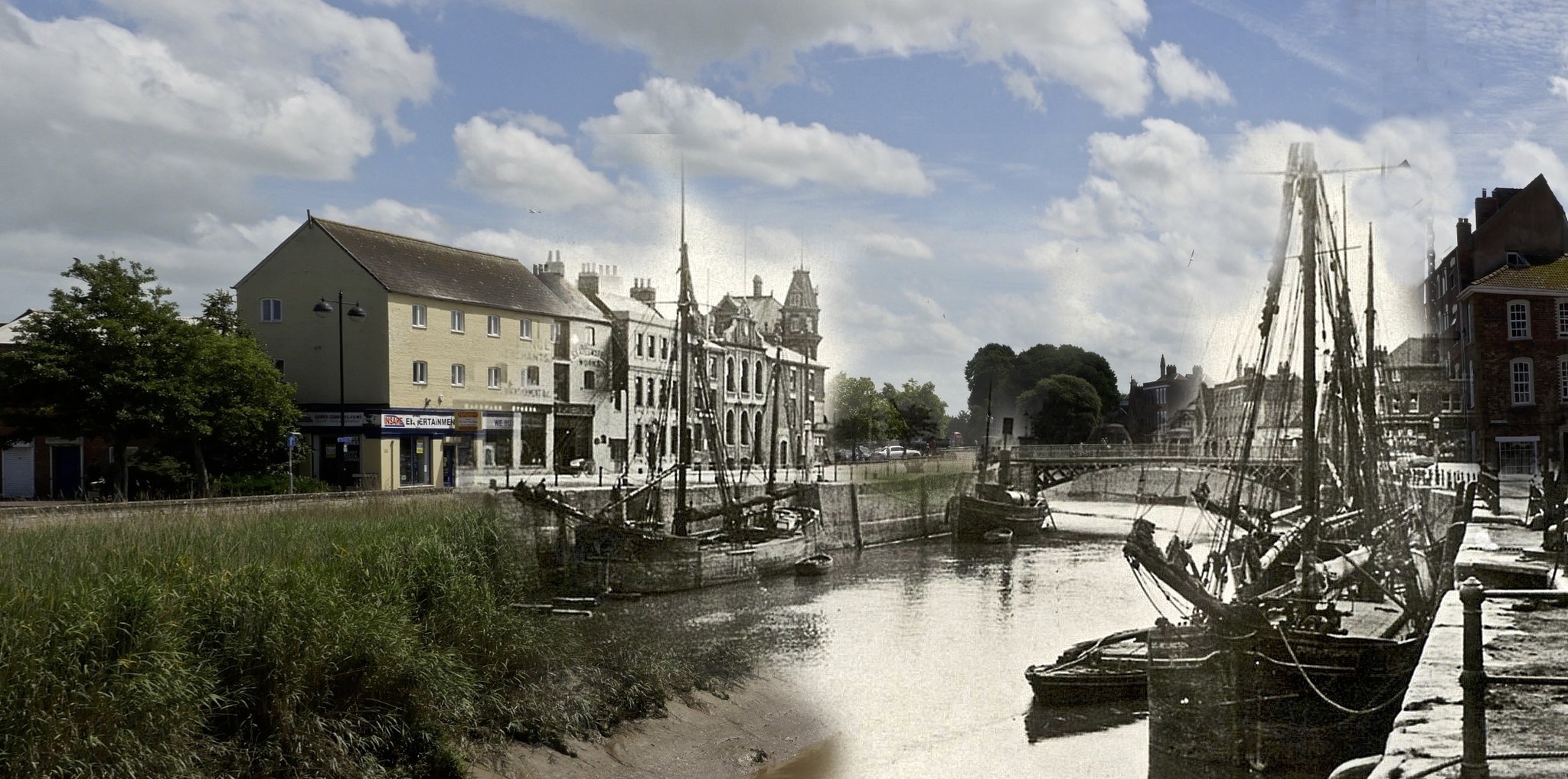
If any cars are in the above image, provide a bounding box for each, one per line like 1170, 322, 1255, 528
833, 449, 871, 462
871, 445, 921, 460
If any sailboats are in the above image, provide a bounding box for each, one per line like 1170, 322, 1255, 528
511, 157, 824, 598
1123, 142, 1446, 778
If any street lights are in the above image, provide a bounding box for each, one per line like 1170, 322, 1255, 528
313, 288, 368, 491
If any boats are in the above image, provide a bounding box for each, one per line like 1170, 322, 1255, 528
944, 376, 1051, 540
1024, 627, 1151, 707
794, 552, 834, 577
